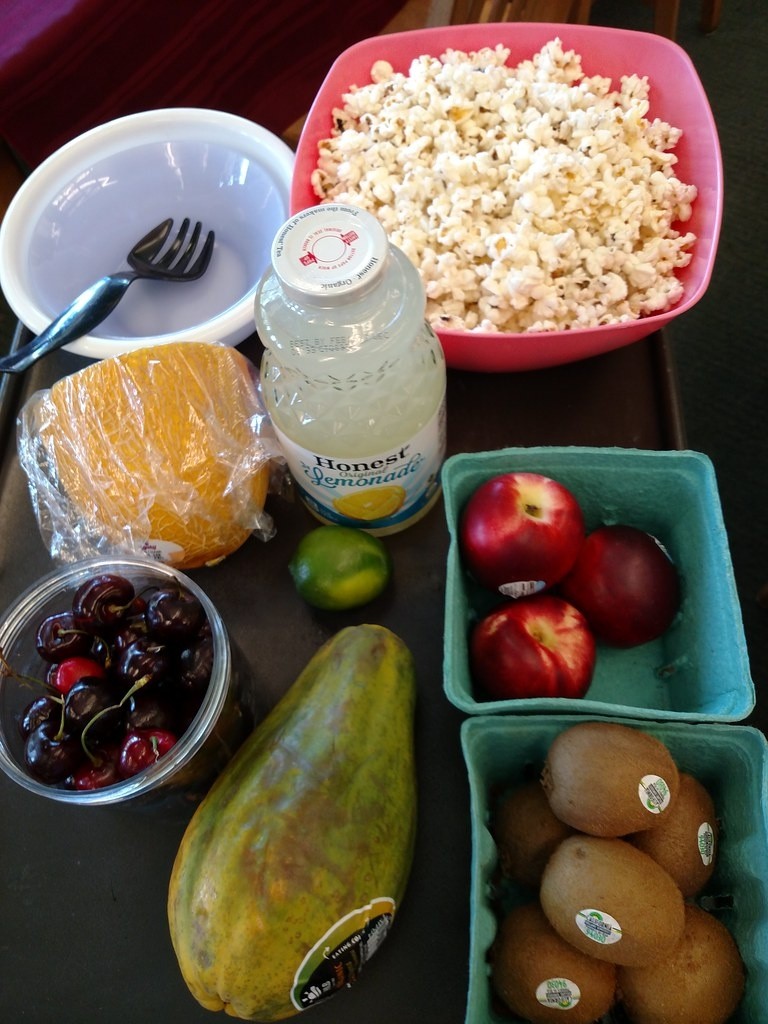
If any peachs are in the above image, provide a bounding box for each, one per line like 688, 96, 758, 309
466, 470, 684, 700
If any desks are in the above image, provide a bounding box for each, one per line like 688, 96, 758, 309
0, 0, 685, 1024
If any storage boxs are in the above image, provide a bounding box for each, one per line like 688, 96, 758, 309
437, 444, 756, 723
293, 24, 726, 373
459, 715, 768, 1024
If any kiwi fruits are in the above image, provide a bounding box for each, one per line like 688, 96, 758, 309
487, 722, 750, 1024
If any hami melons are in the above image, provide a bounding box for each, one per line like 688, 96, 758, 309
30, 342, 270, 570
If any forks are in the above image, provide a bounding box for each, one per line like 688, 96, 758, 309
0, 218, 214, 376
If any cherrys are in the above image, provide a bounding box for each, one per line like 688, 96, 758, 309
0, 569, 214, 791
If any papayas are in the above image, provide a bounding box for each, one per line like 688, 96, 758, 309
171, 621, 416, 1019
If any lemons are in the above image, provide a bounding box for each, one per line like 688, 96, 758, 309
287, 526, 392, 610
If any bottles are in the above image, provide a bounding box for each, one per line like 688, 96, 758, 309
252, 203, 445, 537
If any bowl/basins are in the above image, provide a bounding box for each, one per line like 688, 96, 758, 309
0, 106, 294, 363
288, 23, 725, 371
0, 554, 234, 806
